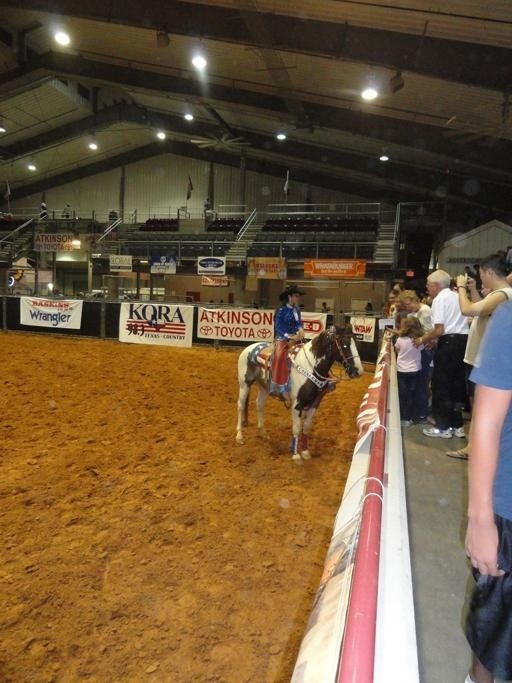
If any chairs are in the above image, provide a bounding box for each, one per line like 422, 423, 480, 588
0, 218, 379, 277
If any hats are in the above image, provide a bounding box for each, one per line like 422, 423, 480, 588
278, 285, 307, 303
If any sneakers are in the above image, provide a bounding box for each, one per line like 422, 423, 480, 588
400, 415, 437, 428
422, 426, 453, 438
449, 426, 466, 438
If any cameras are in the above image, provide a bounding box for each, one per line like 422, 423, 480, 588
449, 278, 470, 293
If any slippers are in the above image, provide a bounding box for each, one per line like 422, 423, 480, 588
446, 449, 469, 459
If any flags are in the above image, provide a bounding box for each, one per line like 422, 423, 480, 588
185, 176, 195, 201
38, 194, 48, 222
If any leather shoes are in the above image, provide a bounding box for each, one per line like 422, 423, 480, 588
281, 392, 291, 408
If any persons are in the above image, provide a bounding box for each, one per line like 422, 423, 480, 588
365, 301, 375, 317
60, 203, 72, 221
320, 301, 329, 313
386, 245, 512, 683
269, 282, 304, 397
202, 198, 212, 217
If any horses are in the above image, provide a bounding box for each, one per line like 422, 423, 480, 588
234, 321, 364, 463
